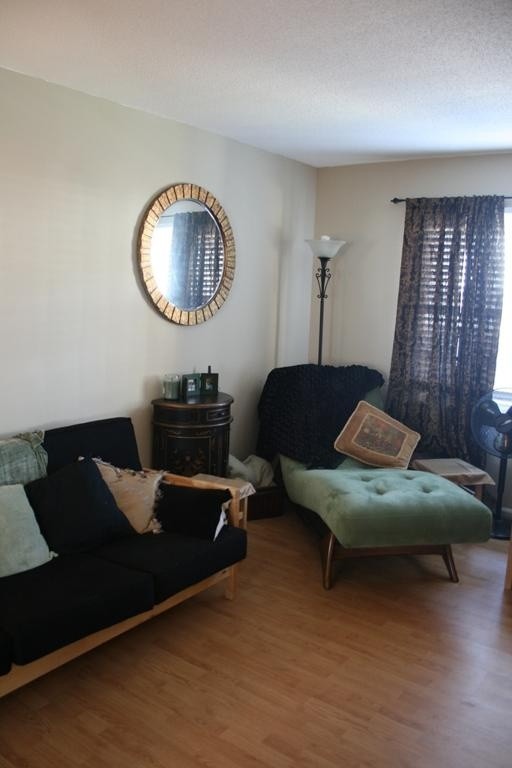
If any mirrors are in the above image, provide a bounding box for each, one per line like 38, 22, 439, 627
137, 180, 239, 327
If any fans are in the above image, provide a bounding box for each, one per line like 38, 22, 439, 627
469, 388, 511, 540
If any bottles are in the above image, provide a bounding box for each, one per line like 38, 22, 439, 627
162, 373, 181, 400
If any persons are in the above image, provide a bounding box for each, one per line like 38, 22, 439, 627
206, 378, 212, 389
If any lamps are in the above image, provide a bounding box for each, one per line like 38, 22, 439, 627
304, 233, 349, 364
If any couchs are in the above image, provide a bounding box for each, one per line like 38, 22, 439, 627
1, 416, 255, 705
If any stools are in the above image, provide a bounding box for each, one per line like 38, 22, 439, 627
412, 458, 496, 501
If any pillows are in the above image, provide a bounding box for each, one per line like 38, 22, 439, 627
1, 455, 172, 578
333, 400, 421, 470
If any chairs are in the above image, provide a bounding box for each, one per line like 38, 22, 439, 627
259, 363, 494, 589
